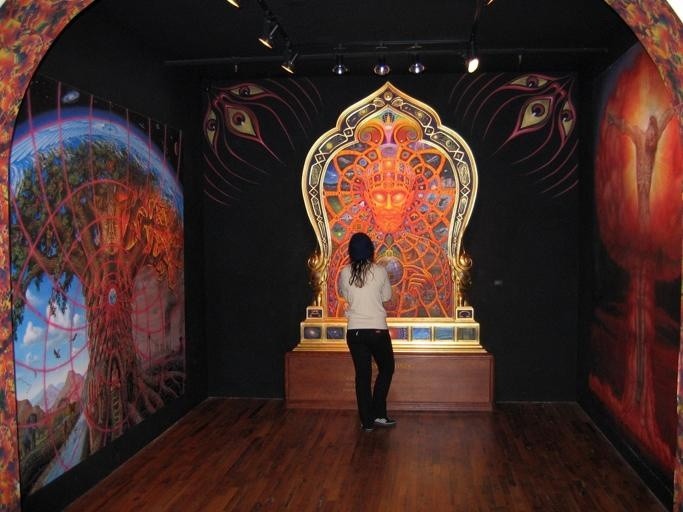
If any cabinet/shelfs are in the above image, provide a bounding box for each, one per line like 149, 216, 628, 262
286, 351, 495, 411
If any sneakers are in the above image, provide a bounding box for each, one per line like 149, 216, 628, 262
373, 417, 396, 426
360, 423, 373, 431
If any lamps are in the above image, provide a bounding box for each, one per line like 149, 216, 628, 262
62, 87, 81, 105
259, 19, 484, 76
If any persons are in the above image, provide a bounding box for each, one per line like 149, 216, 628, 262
606, 106, 675, 240
338, 231, 399, 431
